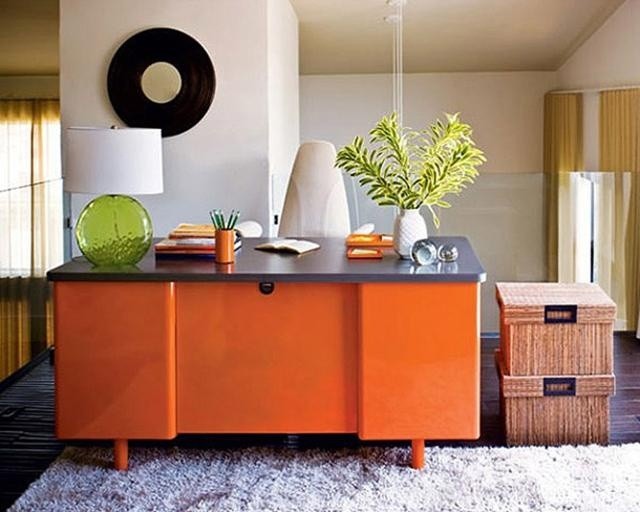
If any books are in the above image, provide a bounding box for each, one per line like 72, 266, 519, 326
254, 240, 321, 254
154, 223, 242, 262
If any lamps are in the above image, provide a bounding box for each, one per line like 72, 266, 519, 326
62, 125, 164, 267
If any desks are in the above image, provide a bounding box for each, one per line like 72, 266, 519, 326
45, 235, 487, 470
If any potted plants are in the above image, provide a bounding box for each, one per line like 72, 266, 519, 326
334, 110, 486, 257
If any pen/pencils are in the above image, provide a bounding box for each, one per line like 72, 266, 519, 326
209, 208, 240, 231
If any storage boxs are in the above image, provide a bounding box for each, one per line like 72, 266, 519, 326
495, 283, 617, 374
492, 346, 615, 445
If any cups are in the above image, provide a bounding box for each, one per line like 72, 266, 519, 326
216, 264, 236, 275
214, 229, 236, 264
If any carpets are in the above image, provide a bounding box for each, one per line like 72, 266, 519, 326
7, 439, 640, 512
